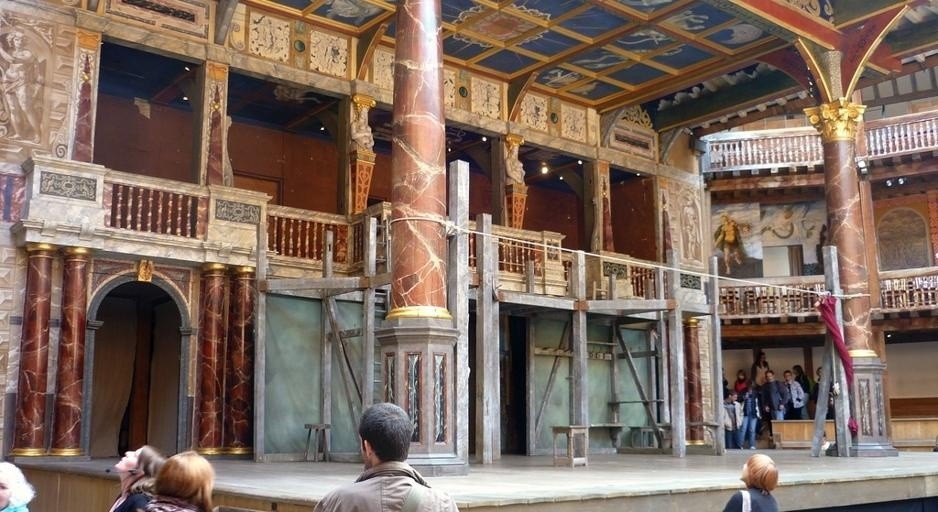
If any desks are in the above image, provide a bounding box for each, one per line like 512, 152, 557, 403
629, 427, 661, 449
550, 425, 590, 468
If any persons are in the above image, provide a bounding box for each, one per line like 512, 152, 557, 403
505, 145, 526, 186
145, 451, 215, 512
350, 109, 375, 152
722, 454, 779, 512
722, 352, 834, 449
683, 200, 699, 259
3, 31, 42, 143
715, 213, 743, 275
312, 403, 459, 512
108, 445, 165, 512
0, 462, 35, 512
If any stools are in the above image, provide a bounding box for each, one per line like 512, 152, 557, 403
304, 423, 333, 461
